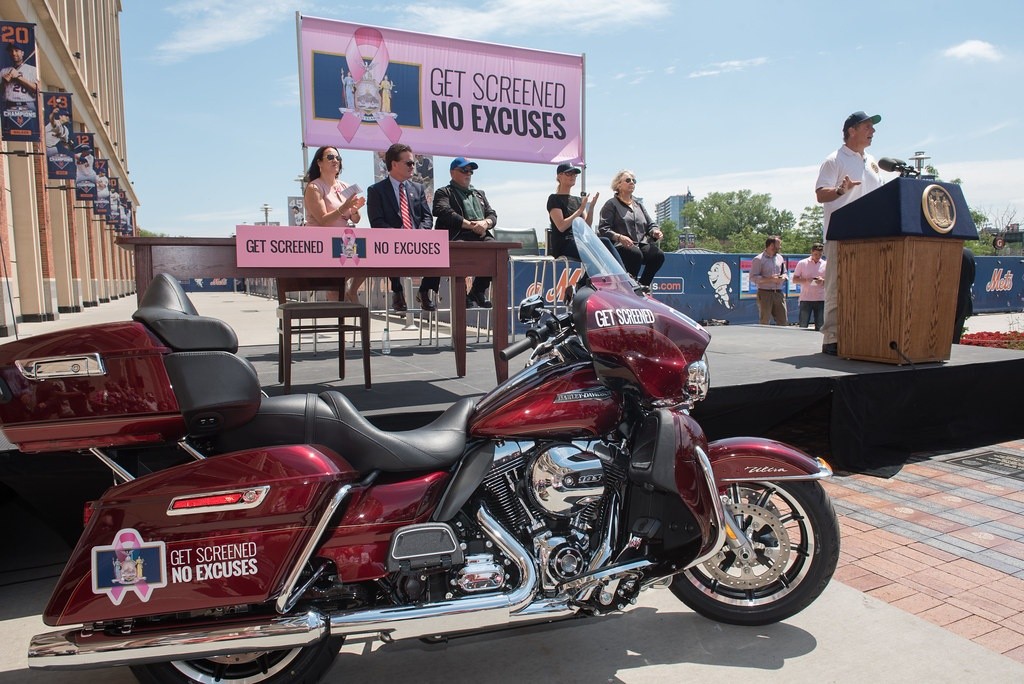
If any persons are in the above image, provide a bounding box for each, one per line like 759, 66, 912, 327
793, 243, 827, 332
0, 42, 38, 136
74, 144, 96, 192
367, 144, 436, 311
414, 153, 433, 187
598, 169, 665, 294
432, 157, 497, 308
15, 376, 175, 420
546, 163, 650, 295
952, 248, 976, 344
815, 111, 889, 357
95, 168, 133, 233
749, 238, 790, 326
292, 206, 304, 226
44, 108, 72, 168
304, 146, 366, 304
377, 151, 393, 180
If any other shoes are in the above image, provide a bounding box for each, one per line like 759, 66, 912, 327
344, 292, 360, 303
822, 342, 837, 356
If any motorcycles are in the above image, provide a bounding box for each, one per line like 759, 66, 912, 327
0, 214, 841, 684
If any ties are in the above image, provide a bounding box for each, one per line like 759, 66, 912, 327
399, 182, 412, 230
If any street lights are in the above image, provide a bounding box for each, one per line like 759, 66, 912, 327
260, 204, 273, 226
908, 151, 932, 179
294, 174, 305, 225
683, 225, 690, 247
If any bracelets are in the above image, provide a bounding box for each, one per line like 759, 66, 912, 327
618, 234, 621, 242
337, 208, 344, 216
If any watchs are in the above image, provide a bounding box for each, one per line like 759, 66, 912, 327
485, 219, 490, 227
835, 187, 843, 196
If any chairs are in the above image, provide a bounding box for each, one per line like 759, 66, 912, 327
276, 277, 372, 395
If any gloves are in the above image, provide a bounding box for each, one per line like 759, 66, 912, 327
0, 66, 21, 82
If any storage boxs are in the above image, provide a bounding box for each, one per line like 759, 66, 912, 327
0, 320, 184, 454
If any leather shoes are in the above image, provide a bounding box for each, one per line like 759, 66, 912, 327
466, 287, 492, 308
416, 290, 435, 311
392, 289, 407, 311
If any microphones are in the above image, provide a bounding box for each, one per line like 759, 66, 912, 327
877, 157, 920, 175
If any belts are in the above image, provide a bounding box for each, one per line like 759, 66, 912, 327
758, 288, 782, 293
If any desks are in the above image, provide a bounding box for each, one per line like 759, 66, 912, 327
115, 237, 523, 387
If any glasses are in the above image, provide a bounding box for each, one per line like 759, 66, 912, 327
400, 160, 415, 167
812, 244, 823, 249
619, 178, 637, 183
563, 171, 577, 177
321, 154, 341, 161
456, 168, 473, 174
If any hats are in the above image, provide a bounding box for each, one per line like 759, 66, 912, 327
844, 111, 881, 132
81, 145, 93, 152
59, 109, 70, 115
7, 42, 24, 51
557, 162, 581, 175
450, 157, 478, 170
99, 167, 107, 173
